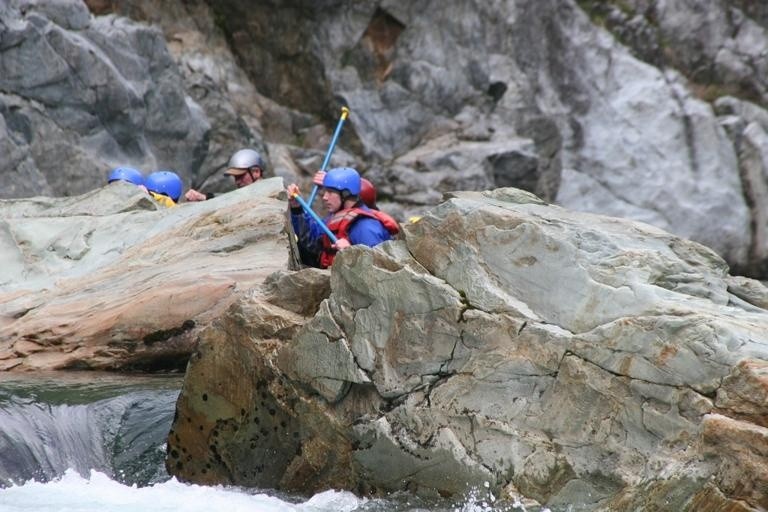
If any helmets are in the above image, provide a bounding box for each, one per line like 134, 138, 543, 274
223, 148, 267, 177
323, 166, 376, 209
146, 170, 184, 202
108, 166, 145, 186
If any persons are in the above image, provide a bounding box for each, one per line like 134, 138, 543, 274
184, 149, 265, 201
287, 167, 400, 269
108, 168, 182, 207
